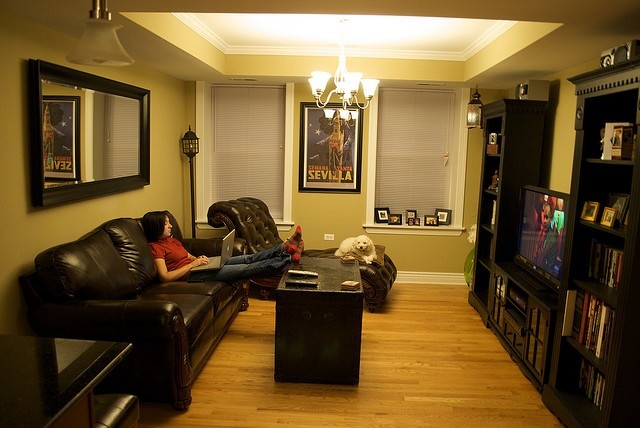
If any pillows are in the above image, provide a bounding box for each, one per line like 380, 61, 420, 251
164, 210, 183, 245
103, 218, 159, 294
35, 229, 138, 300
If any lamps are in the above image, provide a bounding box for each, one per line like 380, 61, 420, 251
308, 20, 380, 110
181, 124, 200, 238
466, 83, 484, 129
65, 0, 135, 67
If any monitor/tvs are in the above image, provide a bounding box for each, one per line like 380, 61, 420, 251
514, 184, 571, 288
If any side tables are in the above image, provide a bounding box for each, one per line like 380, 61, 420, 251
0, 335, 133, 428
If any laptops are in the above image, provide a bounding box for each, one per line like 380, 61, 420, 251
189, 228, 236, 272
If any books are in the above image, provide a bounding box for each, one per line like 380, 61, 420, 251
578, 358, 606, 410
572, 288, 616, 363
587, 237, 624, 290
561, 289, 577, 337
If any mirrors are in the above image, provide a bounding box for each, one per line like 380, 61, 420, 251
28, 58, 150, 207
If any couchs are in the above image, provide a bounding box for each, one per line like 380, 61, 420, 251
18, 237, 251, 410
207, 197, 397, 313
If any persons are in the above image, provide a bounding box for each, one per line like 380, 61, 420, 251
142, 211, 305, 283
534, 202, 558, 276
534, 194, 556, 257
551, 198, 566, 263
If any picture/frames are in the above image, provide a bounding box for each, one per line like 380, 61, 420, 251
375, 208, 390, 222
414, 218, 420, 226
42, 95, 80, 182
435, 209, 452, 225
600, 206, 618, 228
408, 218, 414, 226
406, 210, 416, 223
605, 191, 630, 227
299, 101, 364, 193
388, 214, 402, 225
581, 200, 600, 222
424, 215, 439, 226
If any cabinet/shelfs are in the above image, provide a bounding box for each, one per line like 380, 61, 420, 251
487, 260, 558, 394
542, 56, 640, 428
468, 98, 550, 328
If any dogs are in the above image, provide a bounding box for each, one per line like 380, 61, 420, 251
334, 235, 378, 264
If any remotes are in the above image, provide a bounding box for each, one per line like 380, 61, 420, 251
285, 279, 318, 287
285, 269, 319, 277
